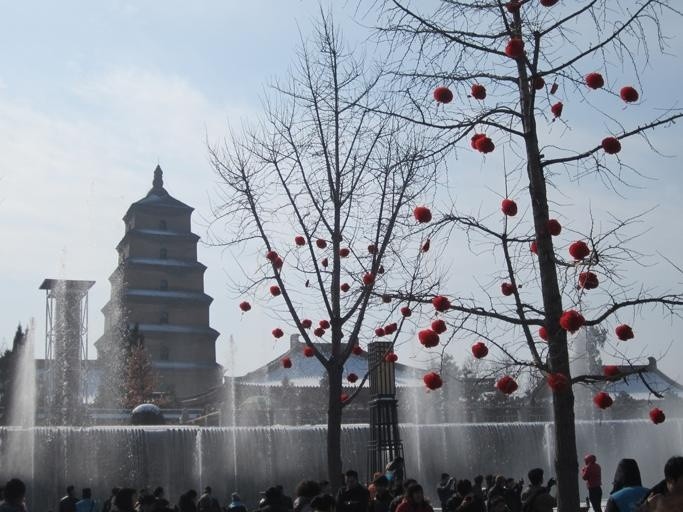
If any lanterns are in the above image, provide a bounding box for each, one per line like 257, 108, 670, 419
404, 199, 666, 424
242, 236, 412, 404
432, 40, 640, 157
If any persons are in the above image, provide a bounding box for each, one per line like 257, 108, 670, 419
1, 452, 683, 511
